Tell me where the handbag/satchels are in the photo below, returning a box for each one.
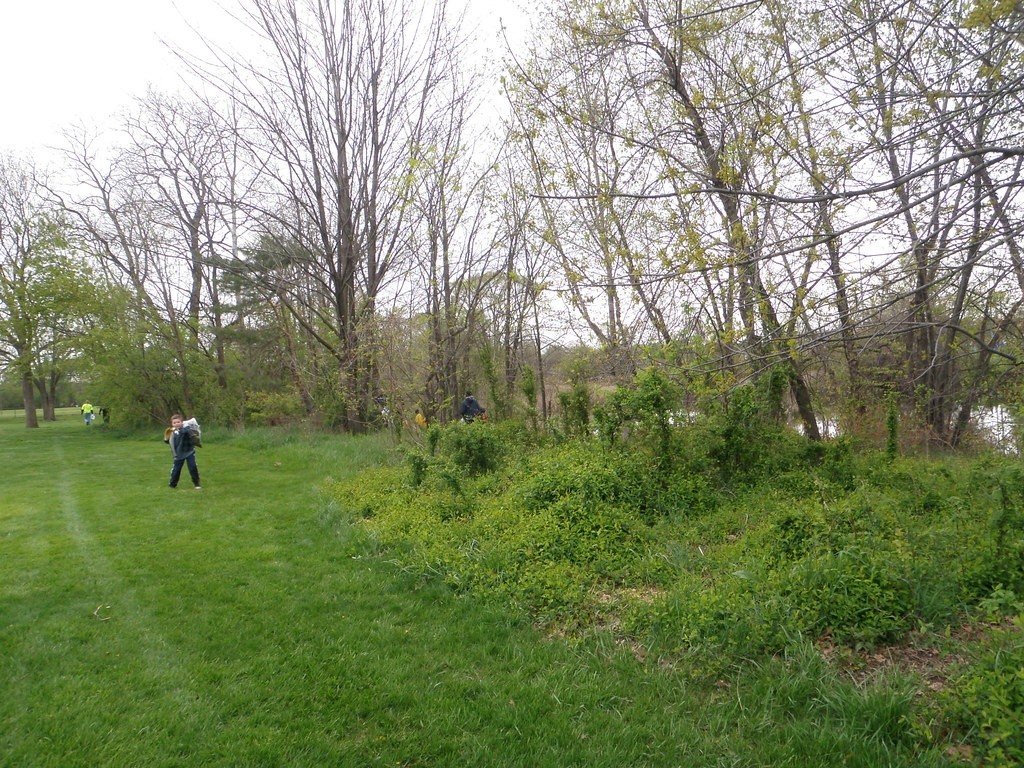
[91,414,95,420]
[183,418,202,448]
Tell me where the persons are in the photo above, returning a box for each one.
[415,408,426,425]
[81,400,95,425]
[98,403,111,423]
[377,389,388,407]
[165,413,202,490]
[459,391,486,424]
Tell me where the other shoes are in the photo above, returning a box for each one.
[87,422,89,425]
[195,486,201,489]
